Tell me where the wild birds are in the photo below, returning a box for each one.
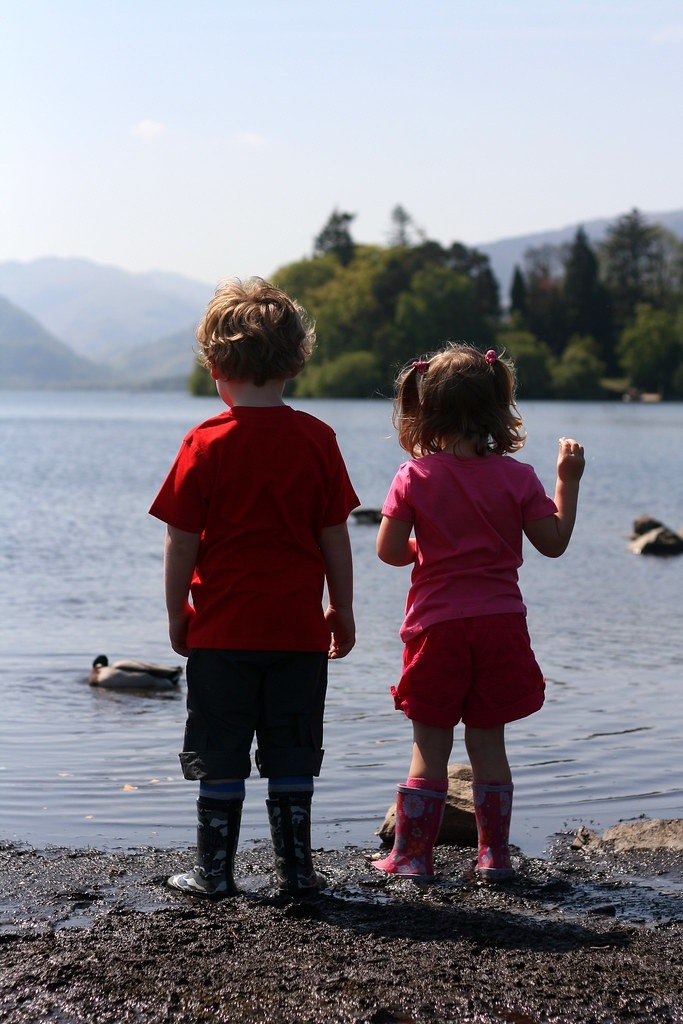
[90,655,184,690]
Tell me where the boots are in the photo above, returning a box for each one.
[372,783,446,882]
[166,799,243,898]
[469,781,514,883]
[266,796,327,893]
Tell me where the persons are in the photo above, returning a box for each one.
[148,278,360,900]
[366,346,585,884]
